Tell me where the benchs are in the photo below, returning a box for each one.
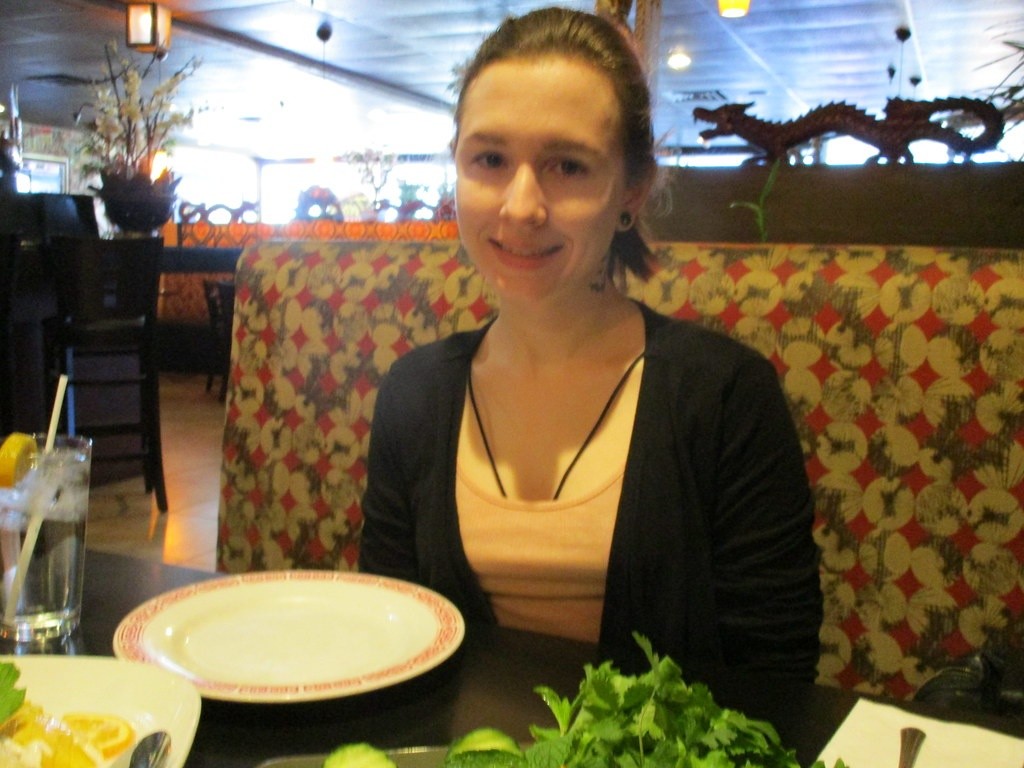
[217,232,1022,717]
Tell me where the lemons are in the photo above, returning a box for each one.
[0,704,133,768]
[0,432,38,489]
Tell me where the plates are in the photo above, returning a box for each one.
[113,568,464,704]
[1,654,202,768]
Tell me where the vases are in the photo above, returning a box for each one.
[86,168,182,237]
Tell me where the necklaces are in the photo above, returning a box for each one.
[464,353,647,505]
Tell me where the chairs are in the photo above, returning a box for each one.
[202,279,234,403]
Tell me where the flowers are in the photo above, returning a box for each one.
[74,37,205,176]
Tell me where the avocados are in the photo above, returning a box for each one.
[444,726,528,768]
[321,741,398,768]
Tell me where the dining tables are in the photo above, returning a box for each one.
[0,550,1024,767]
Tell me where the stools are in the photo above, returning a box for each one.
[44,238,171,512]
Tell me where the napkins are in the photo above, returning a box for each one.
[806,698,1024,768]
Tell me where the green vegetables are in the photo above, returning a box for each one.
[525,630,849,768]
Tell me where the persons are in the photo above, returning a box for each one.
[359,6,825,702]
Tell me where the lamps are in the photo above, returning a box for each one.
[718,1,750,17]
[126,4,172,55]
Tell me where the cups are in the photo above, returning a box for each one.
[0,433,94,641]
